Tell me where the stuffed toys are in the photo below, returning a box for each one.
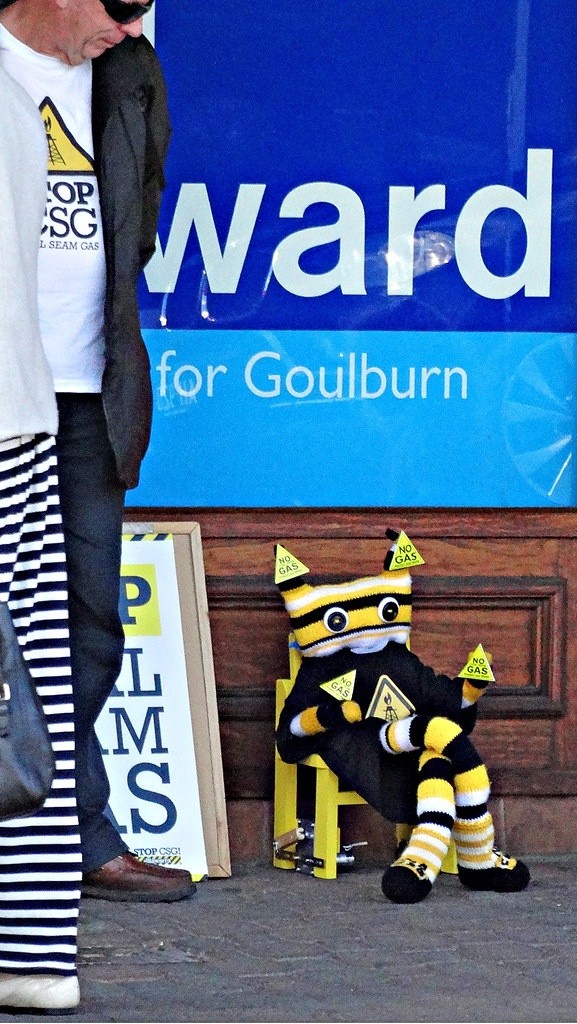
[274,530,533,905]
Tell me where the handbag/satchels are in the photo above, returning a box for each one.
[0,604,56,821]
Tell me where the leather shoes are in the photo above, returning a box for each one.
[80,850,195,905]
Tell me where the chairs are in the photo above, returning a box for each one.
[274,634,459,879]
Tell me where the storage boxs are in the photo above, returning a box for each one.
[94,521,233,882]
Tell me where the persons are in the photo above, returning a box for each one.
[0,48,87,1018]
[0,0,193,899]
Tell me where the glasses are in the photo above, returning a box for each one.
[100,0,152,24]
